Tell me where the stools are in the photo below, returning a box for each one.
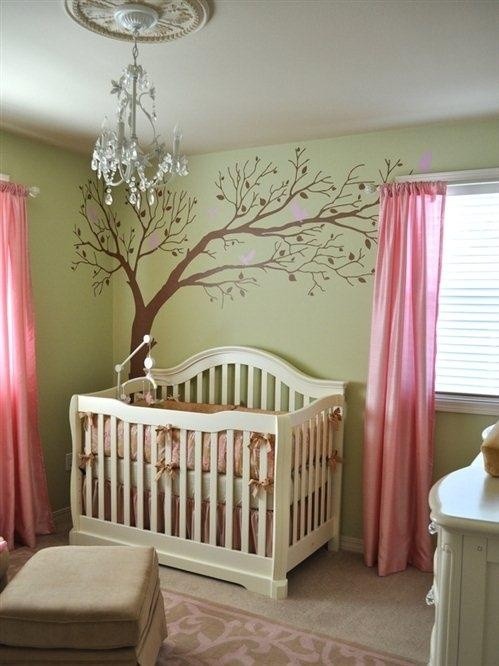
[0,545,169,665]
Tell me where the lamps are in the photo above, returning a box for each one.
[90,4,190,210]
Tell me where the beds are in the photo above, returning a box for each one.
[67,344,351,601]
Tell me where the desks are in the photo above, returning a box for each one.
[422,447,498,666]
